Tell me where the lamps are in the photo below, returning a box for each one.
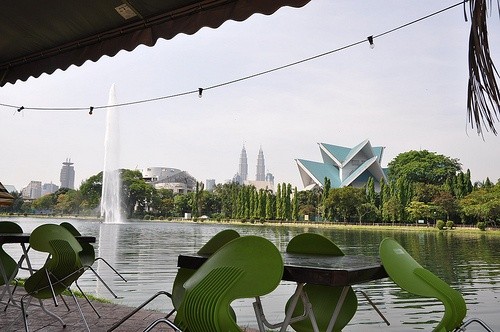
[110,1,143,23]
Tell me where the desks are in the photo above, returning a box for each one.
[177,254,391,332]
[0,232,96,318]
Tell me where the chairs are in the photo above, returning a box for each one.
[56,221,127,318]
[379,237,496,332]
[0,221,24,311]
[281,232,390,331]
[141,235,283,332]
[20,223,118,332]
[107,230,241,332]
